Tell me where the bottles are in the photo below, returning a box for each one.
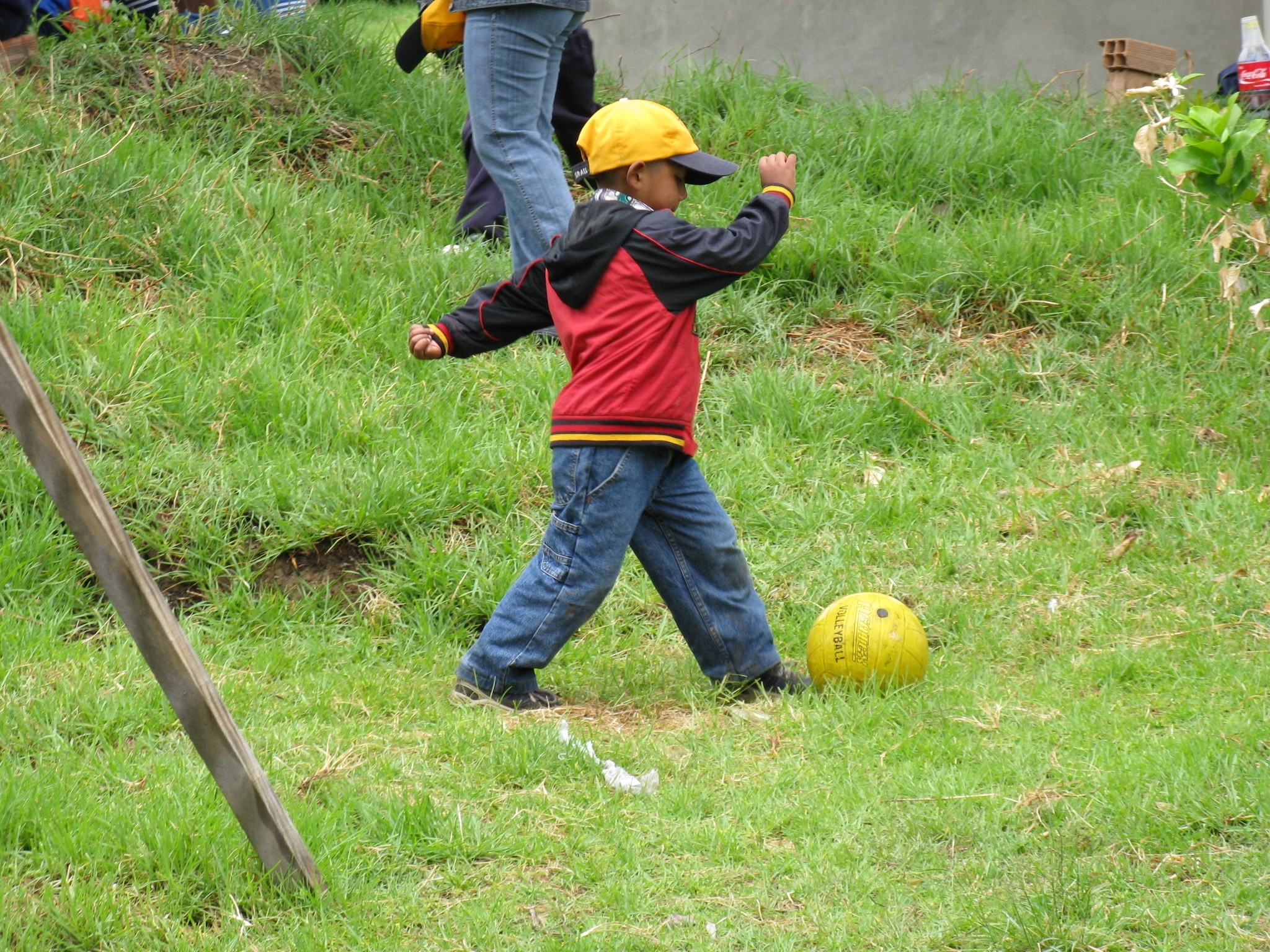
[1238,15,1269,122]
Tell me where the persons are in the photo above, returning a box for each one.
[0,0,421,44]
[407,95,822,715]
[449,0,590,351]
[444,23,600,253]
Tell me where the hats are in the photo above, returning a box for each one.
[571,98,738,185]
[395,0,466,73]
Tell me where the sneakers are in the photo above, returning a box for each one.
[448,676,561,713]
[711,662,812,705]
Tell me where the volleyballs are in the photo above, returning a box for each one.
[807,593,929,690]
